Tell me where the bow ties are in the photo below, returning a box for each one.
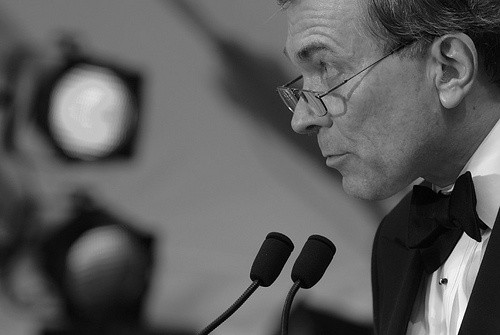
[407,171,482,273]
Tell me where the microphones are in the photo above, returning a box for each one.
[282,235,336,335]
[199,232,294,335]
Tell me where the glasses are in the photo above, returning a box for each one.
[275,37,417,117]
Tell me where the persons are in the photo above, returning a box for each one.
[276,0,500,335]
